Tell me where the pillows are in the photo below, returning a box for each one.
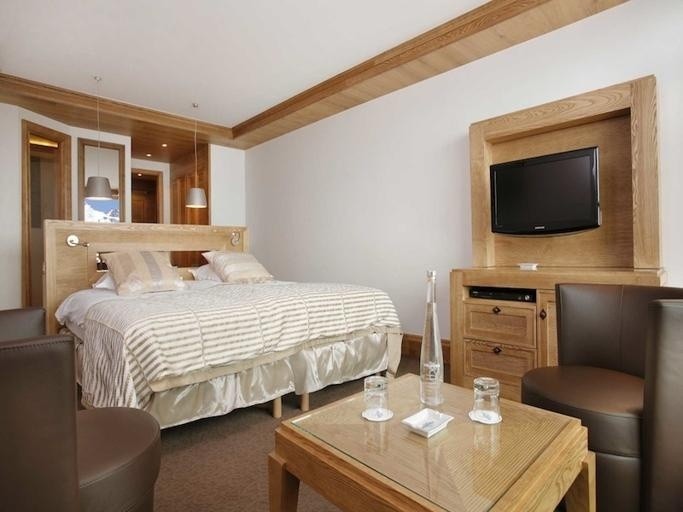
[201,250,274,283]
[99,250,184,297]
[92,271,183,290]
[187,263,273,282]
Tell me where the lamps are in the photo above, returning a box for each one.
[185,104,207,208]
[83,76,113,201]
[231,231,241,246]
[66,234,90,247]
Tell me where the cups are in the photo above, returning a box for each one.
[473,376,500,423]
[363,376,392,419]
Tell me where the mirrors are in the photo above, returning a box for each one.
[20,118,73,307]
[131,168,164,224]
[76,137,125,223]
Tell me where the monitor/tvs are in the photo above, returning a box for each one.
[490,146,600,238]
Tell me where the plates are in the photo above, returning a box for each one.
[400,407,454,437]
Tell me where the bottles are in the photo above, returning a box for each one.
[420,270,447,406]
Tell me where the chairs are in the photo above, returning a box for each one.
[521,283,683,512]
[0,308,161,512]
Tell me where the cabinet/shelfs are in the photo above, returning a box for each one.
[449,266,668,403]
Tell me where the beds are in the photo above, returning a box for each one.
[42,219,388,431]
